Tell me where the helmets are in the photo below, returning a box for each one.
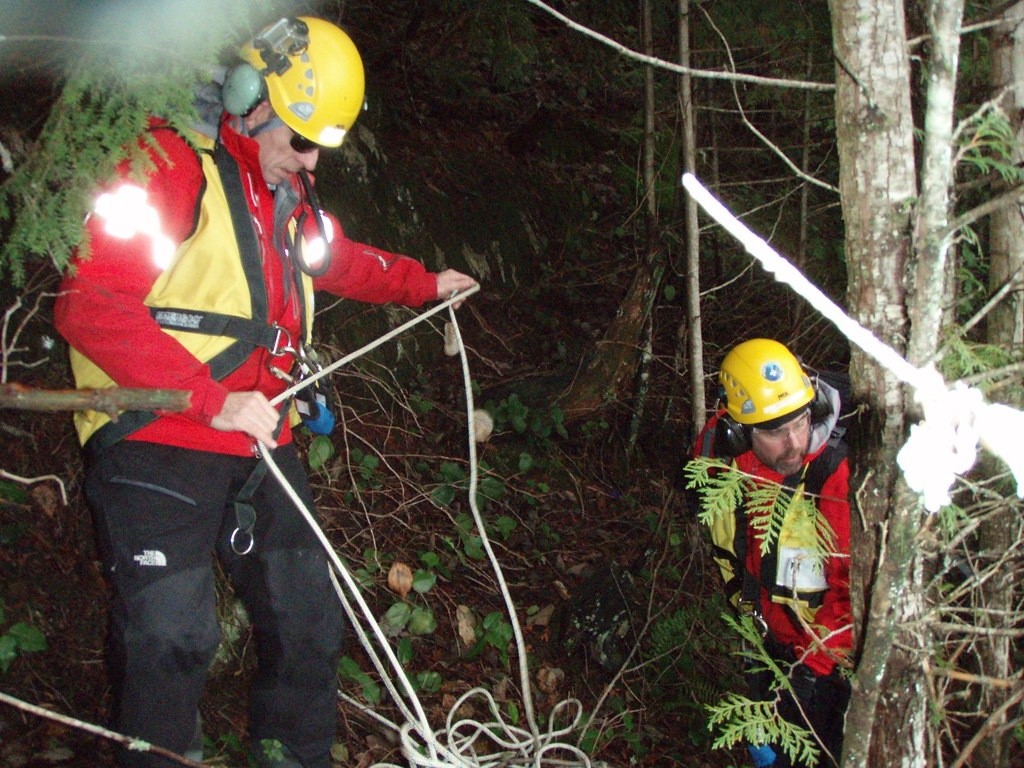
[719,338,815,424]
[237,16,364,148]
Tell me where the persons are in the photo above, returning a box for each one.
[686,336,854,768]
[51,16,478,768]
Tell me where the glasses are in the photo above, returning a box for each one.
[288,125,319,155]
[753,407,811,442]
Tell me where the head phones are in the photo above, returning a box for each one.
[222,65,268,118]
[716,365,831,456]
